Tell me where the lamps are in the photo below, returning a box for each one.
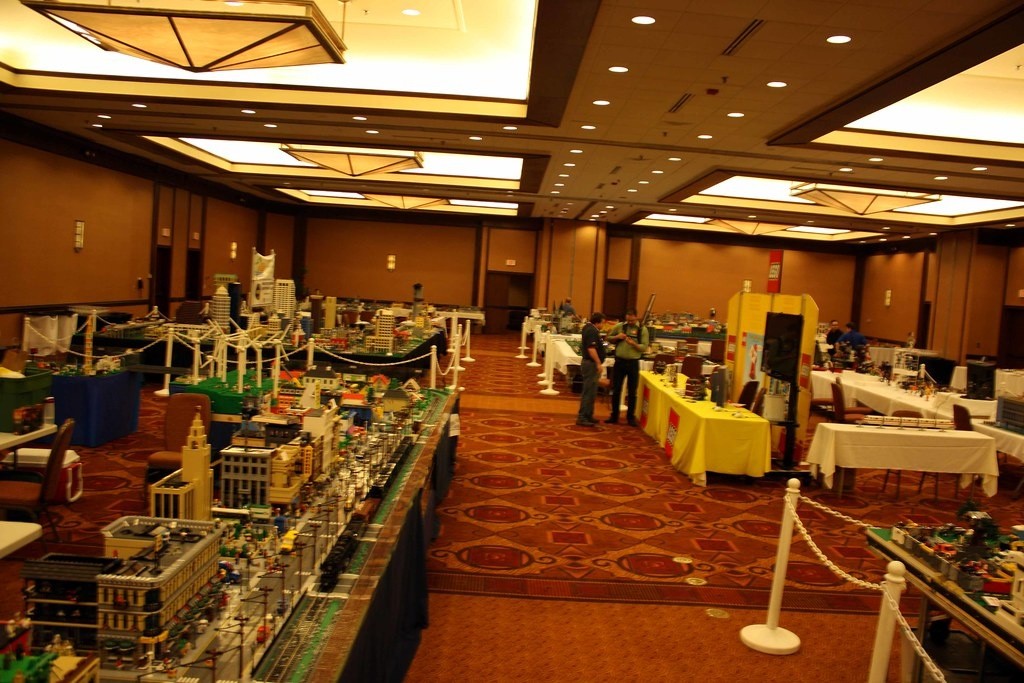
[358,194,449,211]
[742,279,752,292]
[387,254,396,272]
[18,0,349,73]
[278,143,423,178]
[73,220,84,253]
[884,288,892,307]
[790,181,942,215]
[701,218,797,236]
[230,241,237,262]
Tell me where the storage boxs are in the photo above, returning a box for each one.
[830,466,856,493]
[1,449,83,503]
[0,367,54,432]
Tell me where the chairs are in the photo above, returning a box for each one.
[952,404,1001,503]
[528,317,645,393]
[144,393,211,511]
[882,410,939,501]
[830,382,864,425]
[653,354,767,416]
[1,419,76,554]
[808,379,834,420]
[835,376,873,414]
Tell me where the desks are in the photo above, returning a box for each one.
[334,304,486,335]
[864,524,1024,683]
[0,521,43,562]
[805,367,1024,503]
[869,347,939,367]
[0,424,58,471]
[634,371,771,488]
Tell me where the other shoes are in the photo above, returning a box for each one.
[628,419,635,426]
[588,417,599,423]
[576,419,594,426]
[605,417,617,423]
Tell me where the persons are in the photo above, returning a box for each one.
[604,309,649,426]
[563,297,575,316]
[576,313,606,426]
[826,321,866,362]
